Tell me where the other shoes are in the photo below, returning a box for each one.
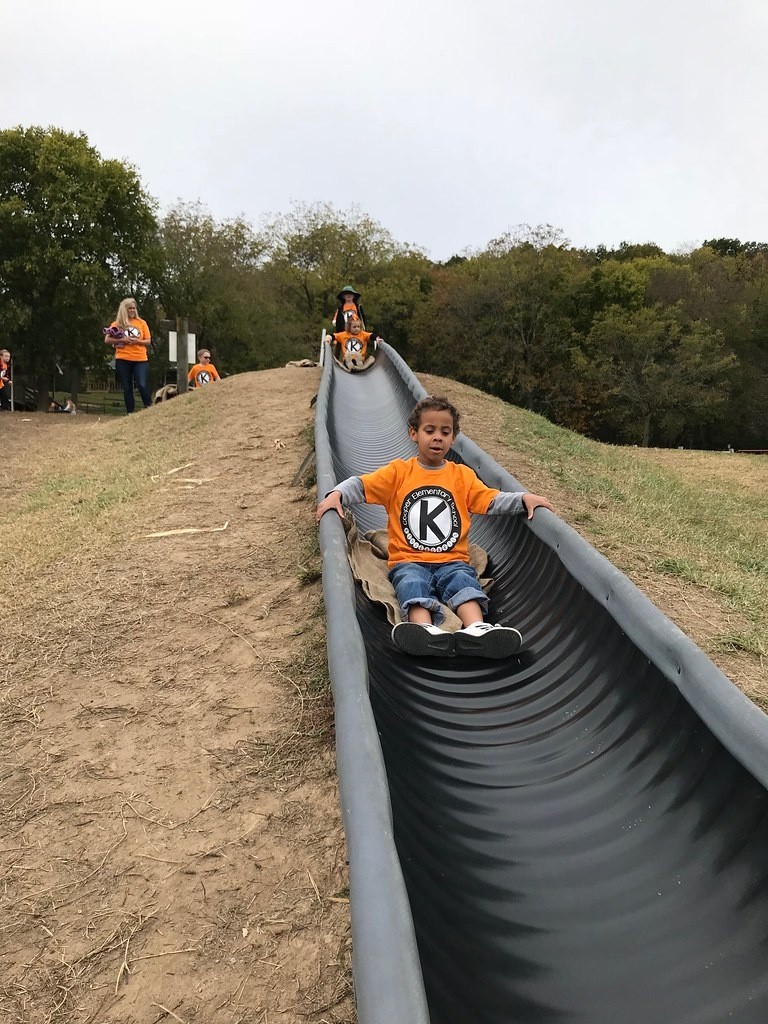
[355,353,363,366]
[346,354,353,368]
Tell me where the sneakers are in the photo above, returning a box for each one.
[391,622,453,657]
[450,622,522,658]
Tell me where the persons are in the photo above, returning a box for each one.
[0,349,13,411]
[104,298,154,416]
[322,286,384,371]
[315,396,556,658]
[188,349,221,387]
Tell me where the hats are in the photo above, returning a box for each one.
[337,286,361,301]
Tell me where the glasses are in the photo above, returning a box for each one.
[202,357,211,359]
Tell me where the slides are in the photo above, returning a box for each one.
[314,339,768,1024]
